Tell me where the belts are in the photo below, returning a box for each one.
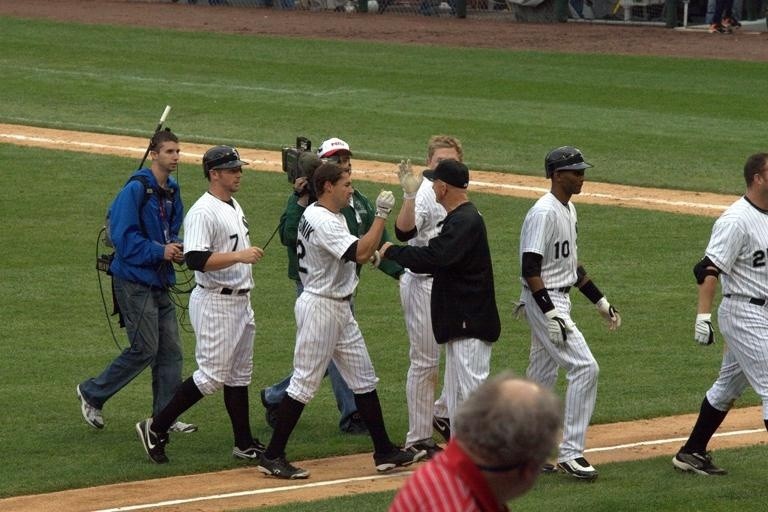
[550,286,576,294]
[345,293,354,301]
[727,292,765,307]
[198,286,248,296]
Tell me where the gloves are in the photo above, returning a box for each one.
[694,312,713,347]
[373,190,395,220]
[595,298,621,332]
[545,309,574,350]
[396,157,424,199]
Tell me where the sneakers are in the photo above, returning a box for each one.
[672,448,728,477]
[559,457,598,480]
[133,418,170,466]
[228,444,268,459]
[344,417,371,434]
[258,389,281,428]
[432,413,452,440]
[543,463,556,475]
[368,447,428,473]
[722,16,743,33]
[405,439,444,463]
[258,453,311,481]
[707,20,731,37]
[165,423,197,439]
[76,383,103,430]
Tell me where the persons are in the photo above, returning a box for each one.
[387,369,566,512]
[708,0,742,35]
[399,135,465,461]
[76,126,198,436]
[670,152,768,476]
[134,144,267,466]
[261,135,405,433]
[257,163,427,479]
[380,159,502,441]
[522,144,623,481]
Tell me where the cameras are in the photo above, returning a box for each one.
[283,137,356,211]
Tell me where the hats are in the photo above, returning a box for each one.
[546,145,594,178]
[202,145,250,173]
[317,137,354,160]
[423,157,469,191]
